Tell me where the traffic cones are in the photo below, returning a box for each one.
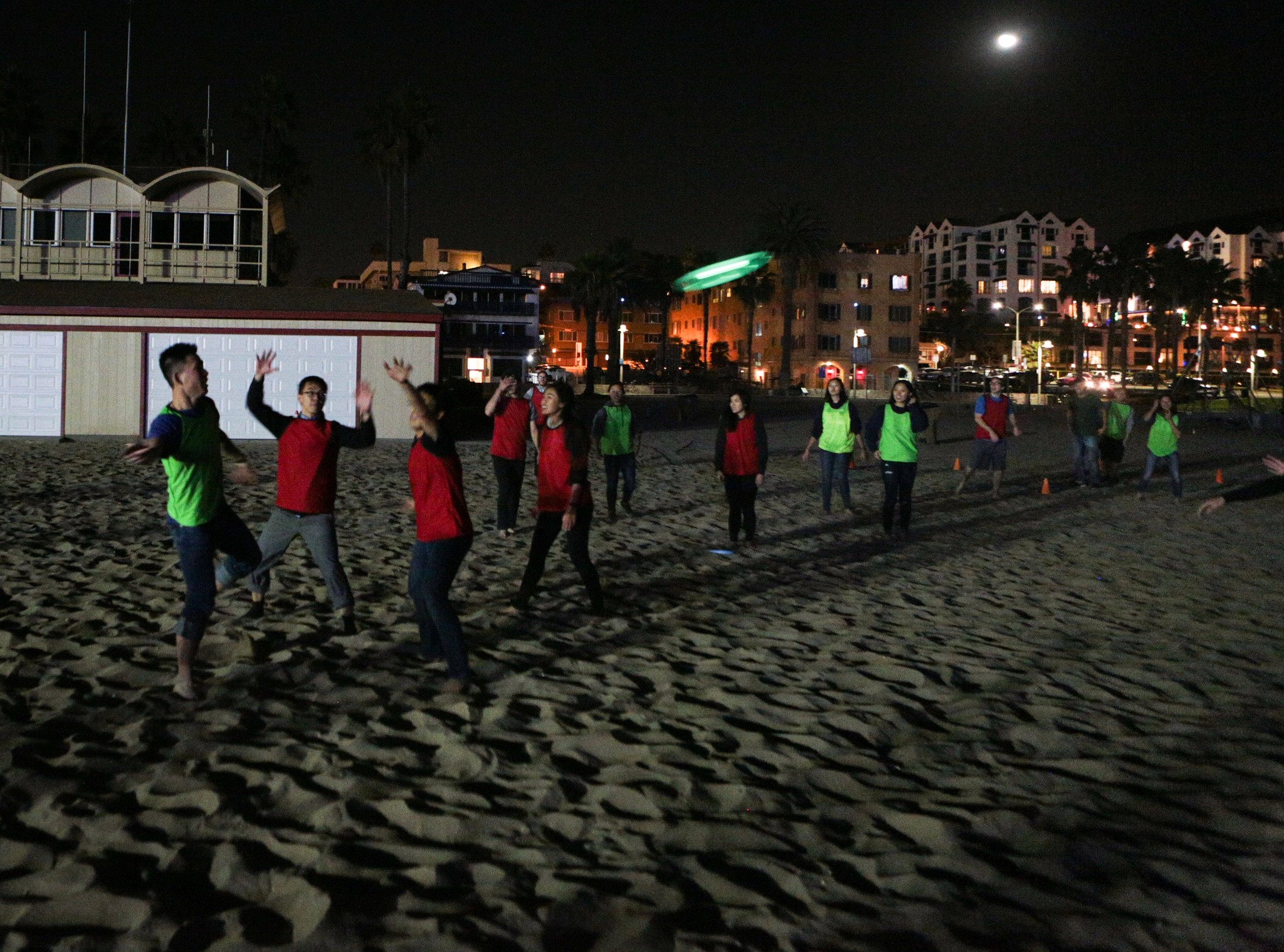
[848,456,857,469]
[1040,479,1051,495]
[953,458,960,471]
[1216,468,1224,484]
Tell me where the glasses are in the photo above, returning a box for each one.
[302,392,325,396]
[538,376,546,378]
[992,382,1001,385]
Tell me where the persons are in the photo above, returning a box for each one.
[800,378,868,515]
[1134,393,1183,509]
[713,389,770,544]
[1066,377,1107,490]
[951,375,1022,500]
[123,342,263,700]
[383,356,474,695]
[1197,453,1284,519]
[865,381,930,538]
[510,381,607,615]
[1103,387,1135,485]
[240,349,377,634]
[592,382,636,525]
[484,372,541,540]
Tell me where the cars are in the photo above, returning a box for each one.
[1056,369,1162,387]
[529,365,575,384]
[918,366,1056,392]
[1165,376,1221,400]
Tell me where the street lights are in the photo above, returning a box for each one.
[1198,305,1223,363]
[1231,301,1242,326]
[992,303,1043,364]
